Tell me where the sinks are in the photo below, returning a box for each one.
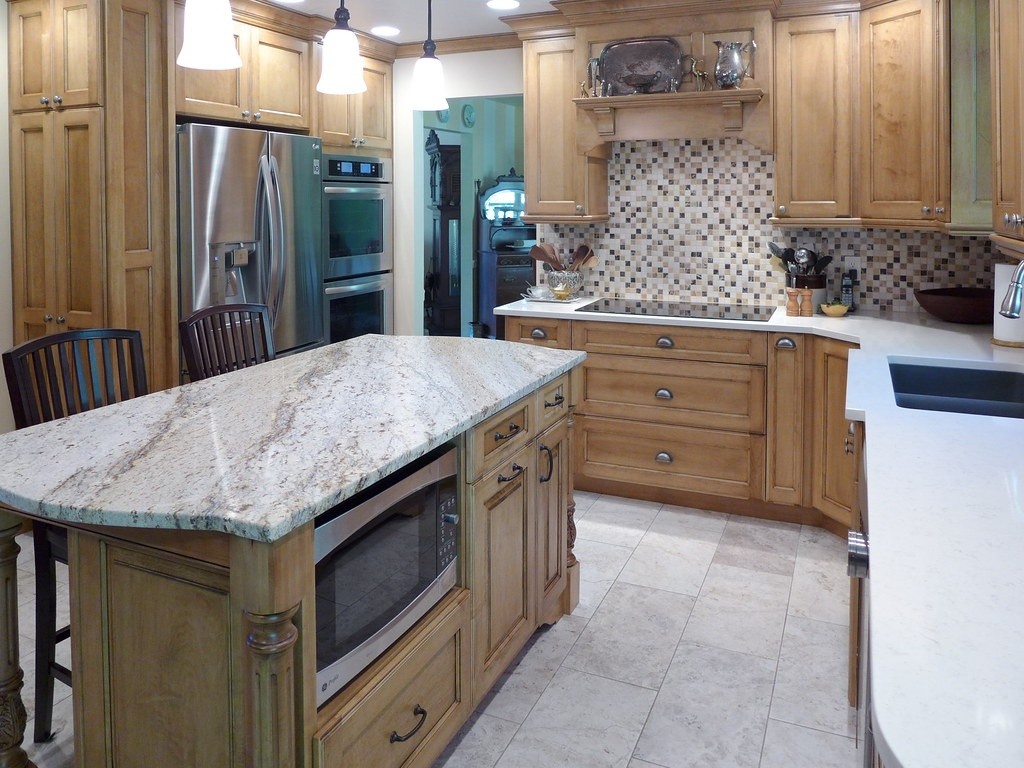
[889,363,1024,419]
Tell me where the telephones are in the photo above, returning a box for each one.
[841,273,857,312]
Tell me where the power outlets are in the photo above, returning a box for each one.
[845,257,862,281]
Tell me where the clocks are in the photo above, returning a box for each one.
[463,105,475,127]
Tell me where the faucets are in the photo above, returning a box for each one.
[999,258,1024,319]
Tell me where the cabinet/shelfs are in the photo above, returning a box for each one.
[425,130,461,337]
[497,0,1024,262]
[309,14,396,156]
[165,1,311,132]
[476,169,537,340]
[67,314,864,768]
[7,0,179,421]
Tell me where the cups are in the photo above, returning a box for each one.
[527,287,547,298]
[785,273,826,313]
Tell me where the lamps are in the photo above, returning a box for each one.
[316,0,367,94]
[176,0,243,70]
[404,0,449,111]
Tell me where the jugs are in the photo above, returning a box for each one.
[713,40,757,89]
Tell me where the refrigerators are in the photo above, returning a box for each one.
[177,122,326,386]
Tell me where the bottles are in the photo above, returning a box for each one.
[843,276,852,307]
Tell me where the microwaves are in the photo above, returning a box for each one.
[313,446,459,709]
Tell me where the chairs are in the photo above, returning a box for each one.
[1,328,147,744]
[178,303,275,382]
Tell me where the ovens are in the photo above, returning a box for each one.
[322,153,394,344]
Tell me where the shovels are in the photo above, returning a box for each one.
[568,243,599,270]
[539,244,560,262]
[528,245,563,270]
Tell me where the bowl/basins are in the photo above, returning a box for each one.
[914,288,994,324]
[820,304,849,317]
[547,271,584,299]
